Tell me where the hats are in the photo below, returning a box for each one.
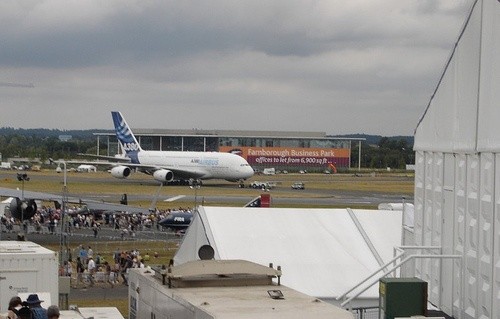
[23,294,44,305]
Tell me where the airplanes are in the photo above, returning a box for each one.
[157,193,270,231]
[50,112,254,186]
[1,185,128,220]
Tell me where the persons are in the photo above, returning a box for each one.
[6,297,23,319]
[17,307,33,319]
[1,199,193,289]
[22,294,48,319]
[46,305,60,319]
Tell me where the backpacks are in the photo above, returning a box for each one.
[8,307,31,319]
